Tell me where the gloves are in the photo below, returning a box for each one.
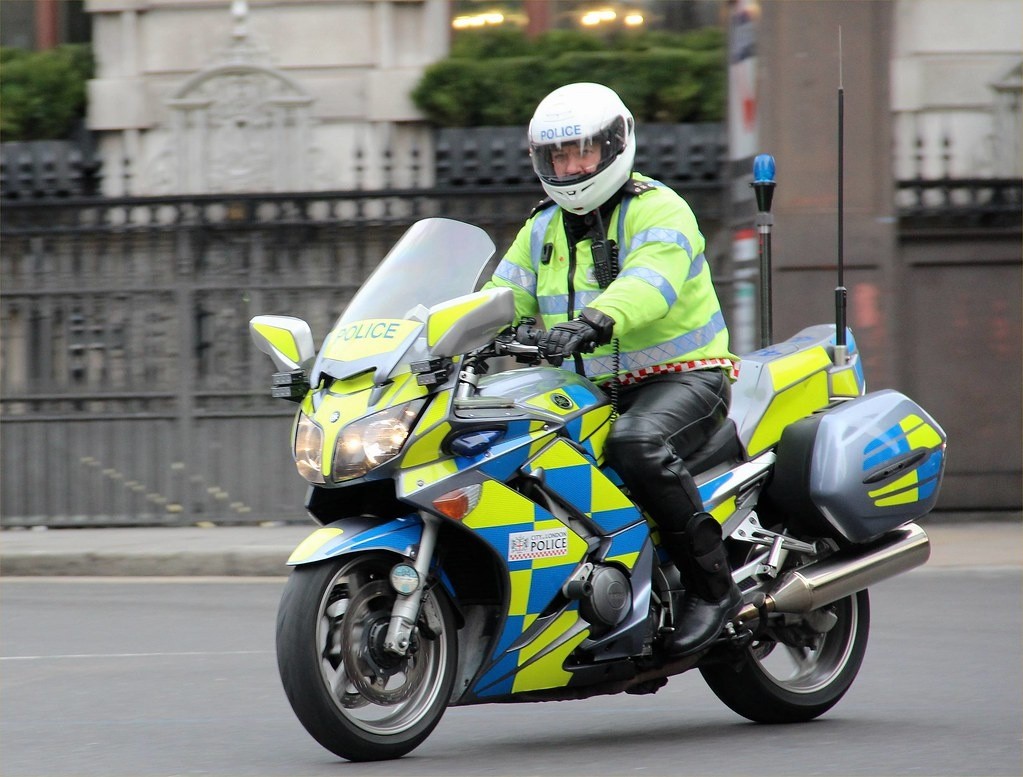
[538,315,597,366]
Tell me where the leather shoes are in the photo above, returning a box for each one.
[670,576,745,655]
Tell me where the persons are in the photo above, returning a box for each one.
[479,82,742,662]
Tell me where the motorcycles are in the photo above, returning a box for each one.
[249,217,947,763]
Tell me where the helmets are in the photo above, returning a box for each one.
[528,83,636,216]
